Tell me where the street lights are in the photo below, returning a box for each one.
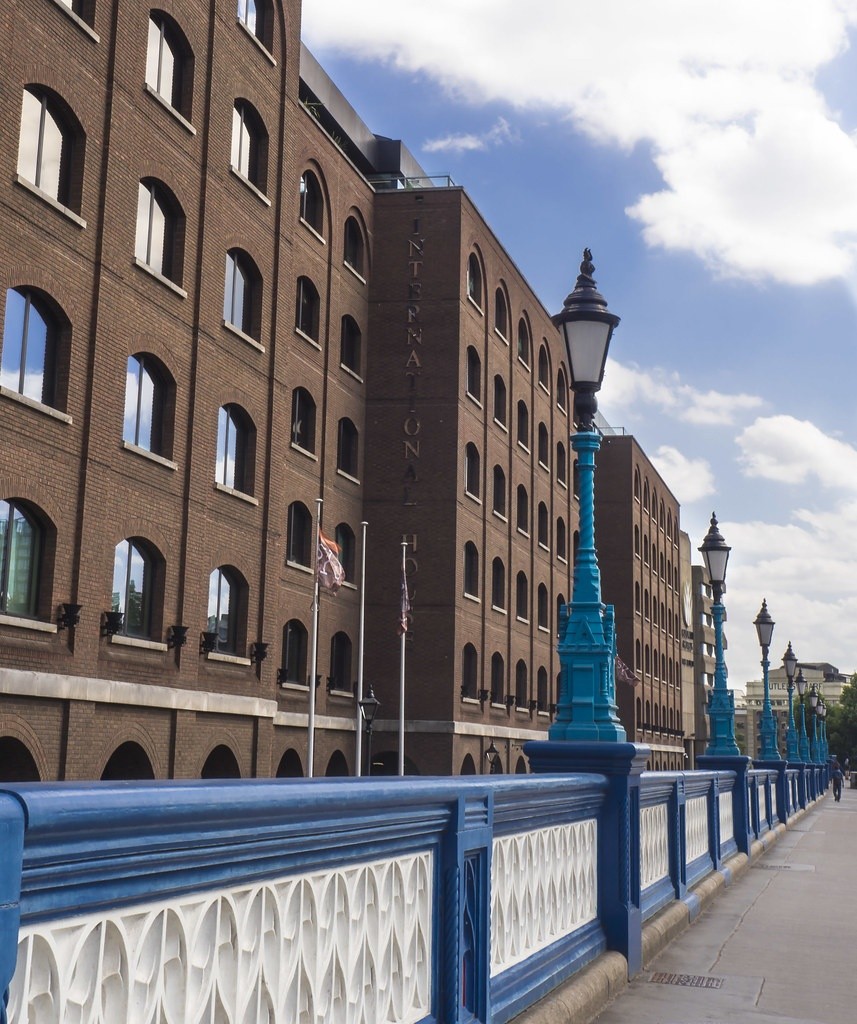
[814,697,827,764]
[752,597,783,760]
[808,684,822,764]
[793,667,813,764]
[697,512,742,757]
[547,247,632,745]
[819,702,830,761]
[781,640,803,762]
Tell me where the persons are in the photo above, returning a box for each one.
[831,758,852,802]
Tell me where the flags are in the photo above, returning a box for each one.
[315,523,346,597]
[614,654,641,687]
[399,555,411,634]
